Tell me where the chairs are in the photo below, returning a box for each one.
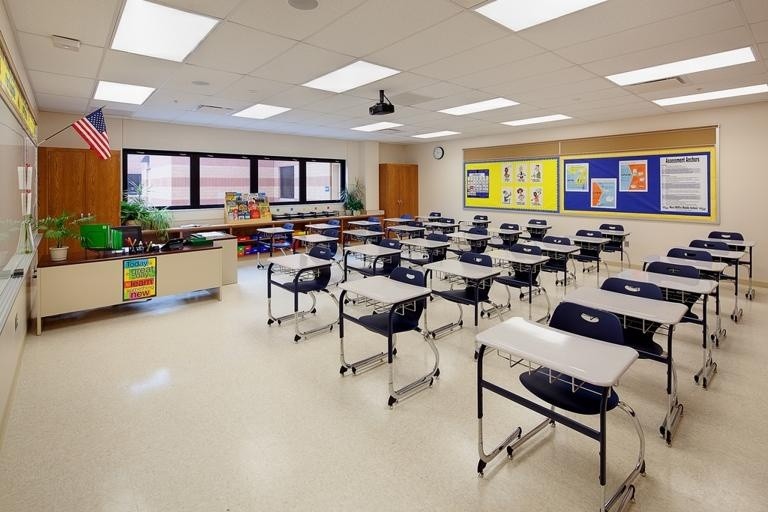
[575,230,603,273]
[359,239,401,306]
[428,212,442,223]
[277,246,340,335]
[506,301,645,512]
[526,220,552,241]
[595,278,677,435]
[352,267,440,409]
[401,233,448,267]
[599,223,630,270]
[383,215,414,238]
[452,228,487,255]
[367,217,380,228]
[645,262,710,382]
[440,253,504,339]
[494,245,551,324]
[472,215,488,227]
[442,218,455,235]
[689,240,739,319]
[667,248,718,339]
[259,223,295,256]
[311,228,344,285]
[539,235,577,286]
[708,231,752,298]
[322,219,341,237]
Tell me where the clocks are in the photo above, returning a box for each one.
[432,147,444,160]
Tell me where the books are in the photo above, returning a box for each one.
[227,192,270,219]
[80,224,122,251]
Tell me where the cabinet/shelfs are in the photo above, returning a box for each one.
[37,146,122,255]
[379,163,419,238]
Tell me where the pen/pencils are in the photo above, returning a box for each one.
[125,237,137,248]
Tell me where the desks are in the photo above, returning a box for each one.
[699,238,755,300]
[387,225,425,238]
[643,256,727,349]
[343,229,385,261]
[596,230,630,269]
[36,243,223,335]
[486,228,522,266]
[399,238,451,267]
[422,254,501,360]
[267,253,340,343]
[458,220,491,232]
[524,224,552,242]
[292,234,340,255]
[474,317,645,512]
[522,240,581,287]
[256,227,295,269]
[666,247,745,322]
[610,269,720,388]
[347,220,381,230]
[560,284,689,447]
[482,249,551,323]
[422,222,460,234]
[567,235,611,285]
[415,215,442,222]
[189,231,238,286]
[338,276,432,405]
[344,244,403,304]
[445,232,491,257]
[305,223,341,235]
[381,217,415,237]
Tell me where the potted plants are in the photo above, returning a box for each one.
[340,191,353,216]
[122,200,149,227]
[349,194,365,216]
[32,209,85,261]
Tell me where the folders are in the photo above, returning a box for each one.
[80,223,122,249]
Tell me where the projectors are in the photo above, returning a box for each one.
[369,102,394,115]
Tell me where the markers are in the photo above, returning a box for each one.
[146,241,153,251]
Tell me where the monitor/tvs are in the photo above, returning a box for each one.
[111,225,141,248]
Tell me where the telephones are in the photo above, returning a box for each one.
[186,233,206,244]
[162,237,184,252]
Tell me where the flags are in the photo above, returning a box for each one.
[73,105,112,160]
